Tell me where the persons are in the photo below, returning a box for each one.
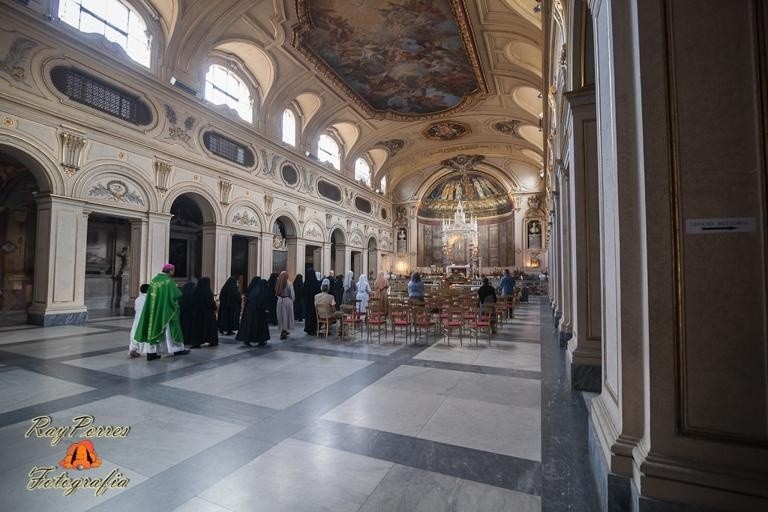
[478,278,496,334]
[500,269,515,318]
[407,272,424,306]
[127,263,390,361]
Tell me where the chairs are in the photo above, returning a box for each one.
[311,277,526,346]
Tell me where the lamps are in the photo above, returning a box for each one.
[438,182,477,249]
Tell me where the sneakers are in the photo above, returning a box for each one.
[174,350,191,356]
[147,353,161,360]
[129,351,140,358]
